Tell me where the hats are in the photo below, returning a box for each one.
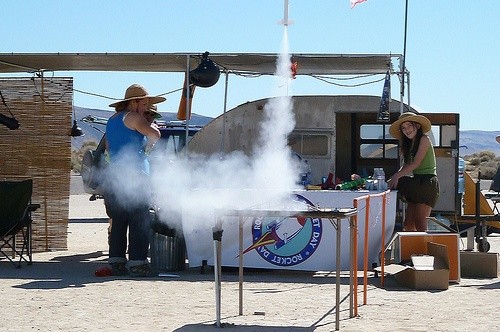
[145,104,162,118]
[389,112,432,139]
[108,85,166,108]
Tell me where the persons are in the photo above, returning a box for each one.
[388,112,440,232]
[105,82,161,277]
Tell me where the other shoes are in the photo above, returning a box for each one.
[110,263,129,276]
[400,259,415,268]
[130,265,159,278]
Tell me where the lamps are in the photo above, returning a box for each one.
[69,105,85,140]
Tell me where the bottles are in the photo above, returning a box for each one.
[458,157,464,194]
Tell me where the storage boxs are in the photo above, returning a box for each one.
[373,241,450,291]
[384,231,461,283]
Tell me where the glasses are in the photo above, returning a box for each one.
[146,112,155,117]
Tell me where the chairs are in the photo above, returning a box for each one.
[0,179,41,269]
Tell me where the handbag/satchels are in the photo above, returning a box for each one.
[82,149,112,191]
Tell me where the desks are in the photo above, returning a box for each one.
[211,207,358,331]
[180,186,399,276]
